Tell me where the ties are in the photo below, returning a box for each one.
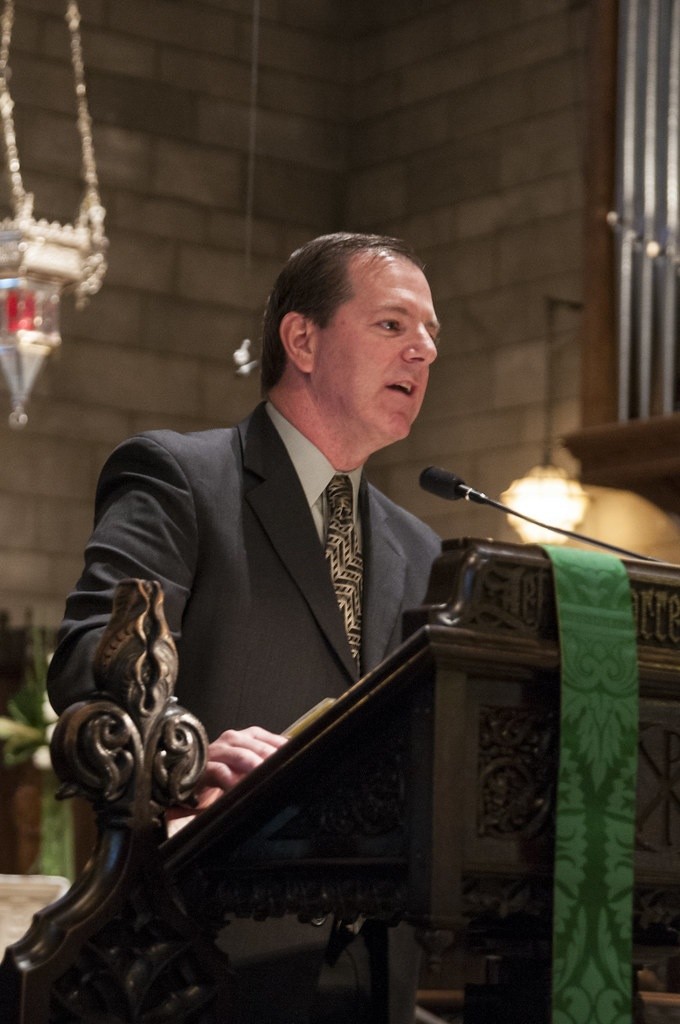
[324,476,364,674]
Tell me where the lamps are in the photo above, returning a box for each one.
[0,0,111,433]
[501,293,592,551]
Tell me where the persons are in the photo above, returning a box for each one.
[45,233,487,1024]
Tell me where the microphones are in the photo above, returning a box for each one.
[420,465,661,563]
